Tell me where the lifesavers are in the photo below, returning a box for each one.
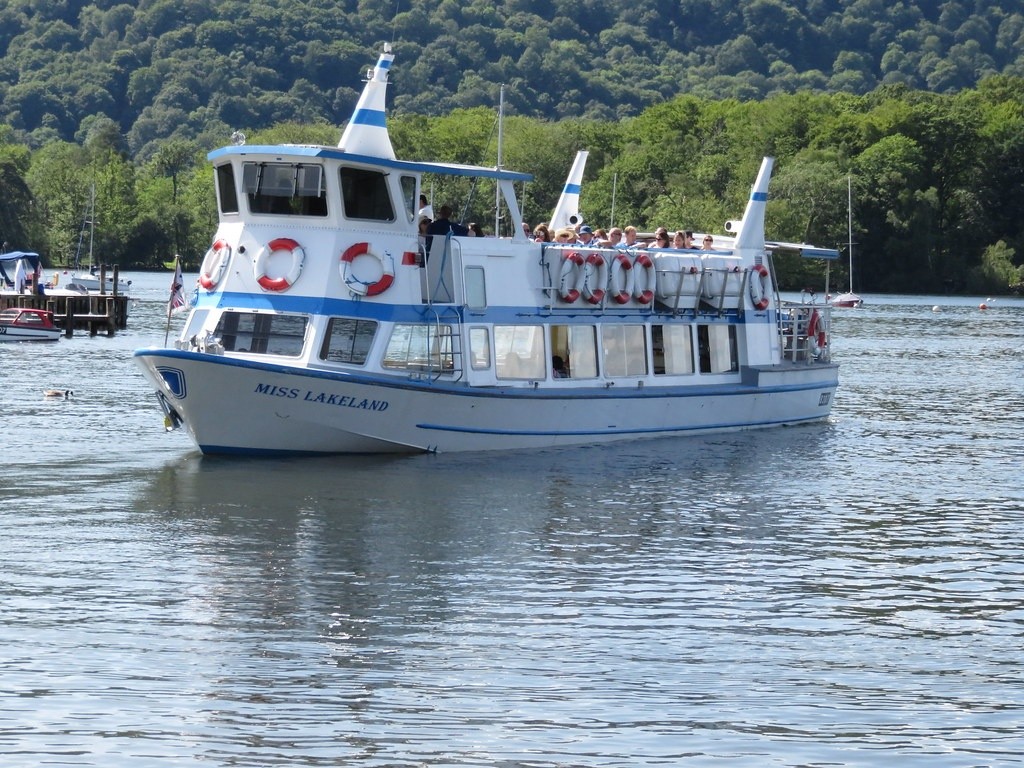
[254,236,306,290]
[559,252,657,304]
[199,238,231,288]
[338,241,396,296]
[807,313,828,355]
[750,265,771,310]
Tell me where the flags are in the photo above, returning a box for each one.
[168,261,190,318]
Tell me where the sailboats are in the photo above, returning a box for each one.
[833,175,864,307]
[70,183,133,292]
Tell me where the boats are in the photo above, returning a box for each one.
[0,251,55,291]
[0,307,61,342]
[130,42,840,464]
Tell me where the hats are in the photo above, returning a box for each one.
[579,226,591,235]
[437,205,452,215]
[555,228,573,241]
[686,232,694,240]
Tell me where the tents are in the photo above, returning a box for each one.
[0,251,40,288]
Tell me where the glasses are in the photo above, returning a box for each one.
[612,232,622,236]
[593,235,601,239]
[655,237,663,241]
[704,239,713,242]
[524,229,529,232]
[561,234,569,237]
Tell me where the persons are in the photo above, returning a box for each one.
[14,259,27,294]
[519,223,717,251]
[419,194,485,238]
[498,352,570,378]
